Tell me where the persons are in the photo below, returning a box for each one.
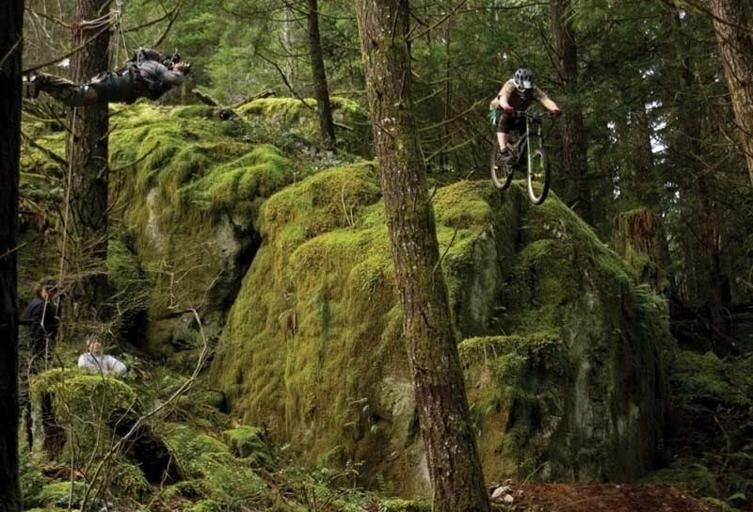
[489,67,561,167]
[78,336,127,378]
[23,284,60,368]
[23,48,190,106]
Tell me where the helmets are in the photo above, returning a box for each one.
[513,68,533,89]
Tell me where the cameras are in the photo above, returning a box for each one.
[179,61,194,71]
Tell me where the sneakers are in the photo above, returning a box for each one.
[22,72,40,98]
[500,149,513,161]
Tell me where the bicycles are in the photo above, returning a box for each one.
[487,107,561,205]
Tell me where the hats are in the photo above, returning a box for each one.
[40,276,56,288]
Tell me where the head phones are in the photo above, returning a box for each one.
[163,47,179,69]
